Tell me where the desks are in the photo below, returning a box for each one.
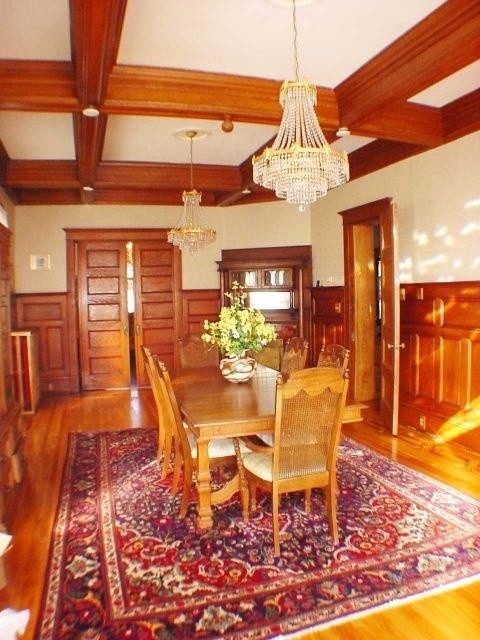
[167,361,368,531]
[10,325,40,415]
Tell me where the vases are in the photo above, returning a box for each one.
[219,356,258,385]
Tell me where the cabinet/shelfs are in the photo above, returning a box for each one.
[215,259,305,349]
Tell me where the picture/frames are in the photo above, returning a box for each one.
[30,254,51,270]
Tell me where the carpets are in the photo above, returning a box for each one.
[33,427,480,640]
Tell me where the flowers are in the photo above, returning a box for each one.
[201,281,279,360]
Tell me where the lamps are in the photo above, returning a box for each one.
[167,131,217,255]
[252,0,350,210]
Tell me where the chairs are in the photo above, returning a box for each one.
[280,337,310,381]
[237,367,349,557]
[140,344,188,465]
[316,344,351,368]
[174,336,219,371]
[151,355,252,519]
